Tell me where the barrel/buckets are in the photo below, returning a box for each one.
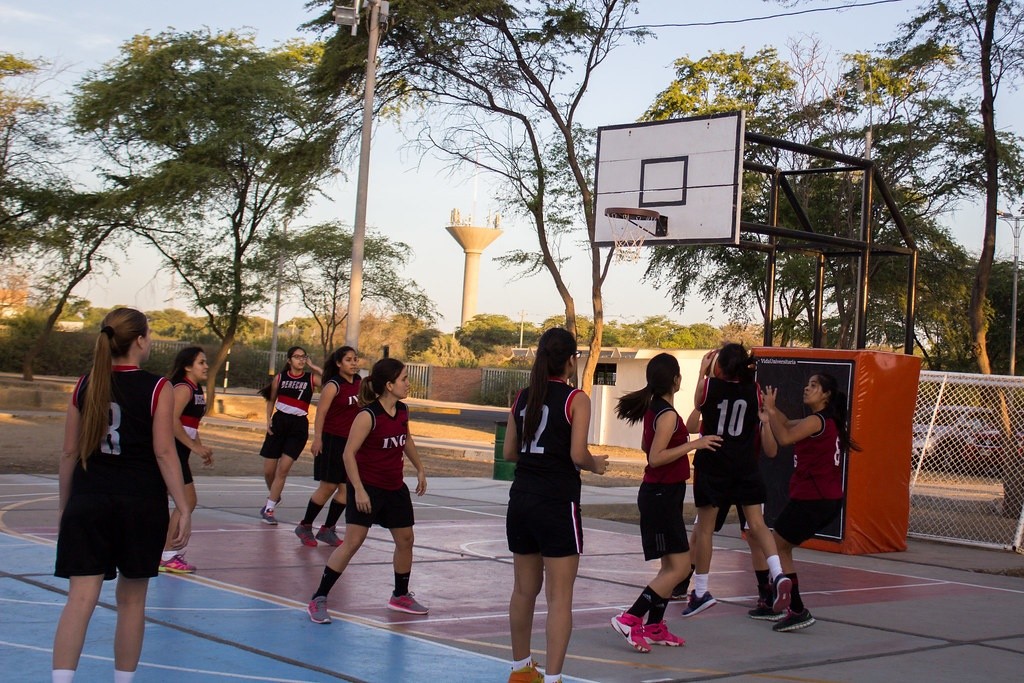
[492,421,517,480]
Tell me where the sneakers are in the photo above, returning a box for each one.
[263,509,278,525]
[772,608,816,632]
[260,497,282,515]
[295,520,318,546]
[642,620,685,647]
[387,591,429,614]
[671,588,686,600]
[612,610,650,652]
[772,574,792,613]
[682,589,716,617]
[747,603,784,621]
[306,593,332,623]
[508,662,544,683]
[158,551,197,573]
[315,525,342,546]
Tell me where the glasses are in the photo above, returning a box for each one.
[574,350,582,358]
[292,354,308,360]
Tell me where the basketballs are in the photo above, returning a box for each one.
[704,349,727,380]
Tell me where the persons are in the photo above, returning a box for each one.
[295,346,429,623]
[256,347,323,525]
[502,328,610,683]
[52,308,214,683]
[611,343,865,652]
